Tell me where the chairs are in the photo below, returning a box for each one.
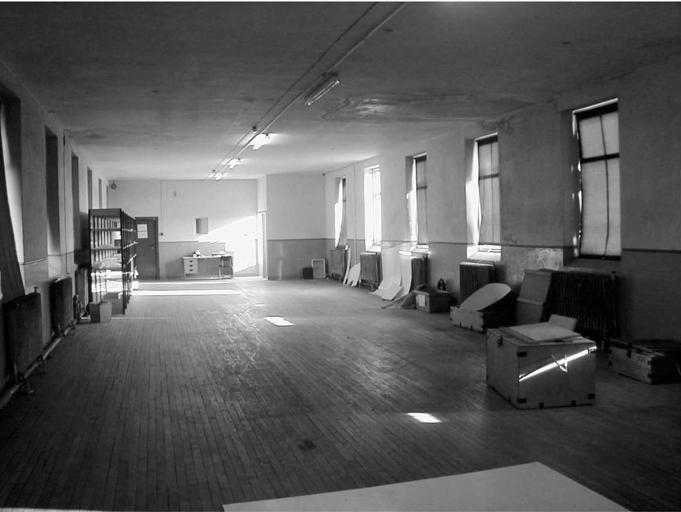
[216,256,233,280]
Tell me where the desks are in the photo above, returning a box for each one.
[182,256,232,281]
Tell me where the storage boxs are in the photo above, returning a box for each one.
[447,303,486,332]
[414,287,451,312]
[88,301,113,324]
[517,268,565,325]
[607,341,678,383]
[482,327,598,410]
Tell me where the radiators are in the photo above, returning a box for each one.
[457,261,498,305]
[1,293,46,392]
[76,266,91,314]
[411,256,428,289]
[541,264,620,346]
[358,252,380,292]
[327,249,349,280]
[48,274,77,340]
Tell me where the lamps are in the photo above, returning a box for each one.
[228,156,240,170]
[303,70,339,107]
[213,172,225,181]
[252,133,273,152]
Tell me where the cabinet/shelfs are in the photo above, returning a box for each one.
[88,204,138,314]
